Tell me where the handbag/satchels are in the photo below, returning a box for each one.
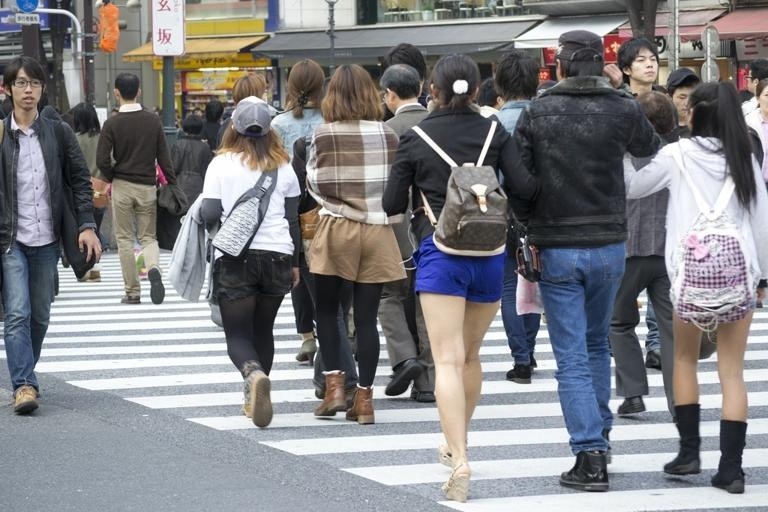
[515,235,543,284]
[211,156,278,262]
[89,177,110,210]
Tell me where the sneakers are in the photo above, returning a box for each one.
[410,385,437,404]
[148,267,165,305]
[644,348,662,371]
[120,295,141,304]
[314,384,358,411]
[616,395,646,414]
[506,351,537,385]
[13,385,38,413]
[384,359,423,395]
[77,263,101,282]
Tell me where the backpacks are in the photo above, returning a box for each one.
[410,120,510,258]
[668,143,762,333]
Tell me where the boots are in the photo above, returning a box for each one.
[346,382,375,426]
[663,403,703,477]
[710,419,747,495]
[239,359,274,428]
[559,426,614,492]
[313,369,349,417]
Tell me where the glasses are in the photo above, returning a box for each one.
[9,78,43,89]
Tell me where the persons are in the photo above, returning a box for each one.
[0,54,102,414]
[489,28,768,494]
[201,42,543,503]
[168,100,227,216]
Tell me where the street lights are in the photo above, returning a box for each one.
[94,0,144,119]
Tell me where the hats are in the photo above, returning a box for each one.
[554,30,604,63]
[665,68,700,92]
[230,95,273,138]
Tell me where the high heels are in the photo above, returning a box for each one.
[438,445,468,469]
[440,462,471,503]
[295,338,317,366]
